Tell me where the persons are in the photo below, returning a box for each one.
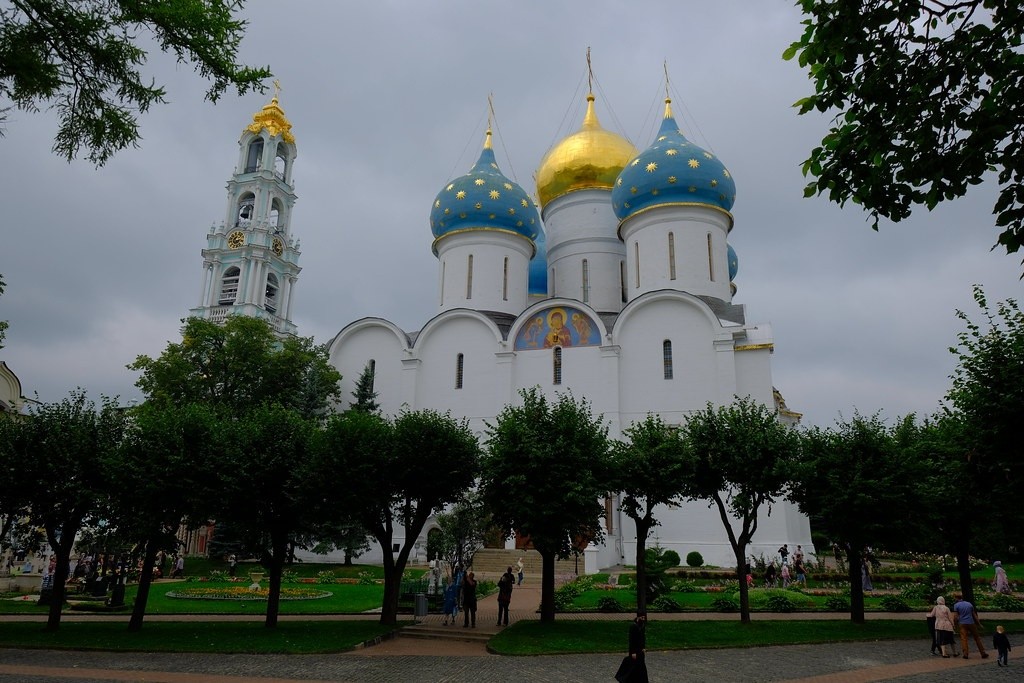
[21,537,184,595]
[501,566,516,611]
[228,554,236,579]
[993,625,1011,666]
[860,557,874,598]
[833,543,846,573]
[462,572,477,629]
[496,573,513,627]
[927,607,942,656]
[992,561,1012,596]
[517,558,524,585]
[926,596,960,659]
[443,577,457,626]
[951,591,989,659]
[745,543,807,591]
[453,564,467,611]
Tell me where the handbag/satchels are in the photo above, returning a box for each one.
[514,565,520,571]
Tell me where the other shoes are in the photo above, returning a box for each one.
[471,625,475,628]
[496,623,501,626]
[502,623,508,626]
[442,621,447,626]
[450,620,455,625]
[463,625,468,628]
